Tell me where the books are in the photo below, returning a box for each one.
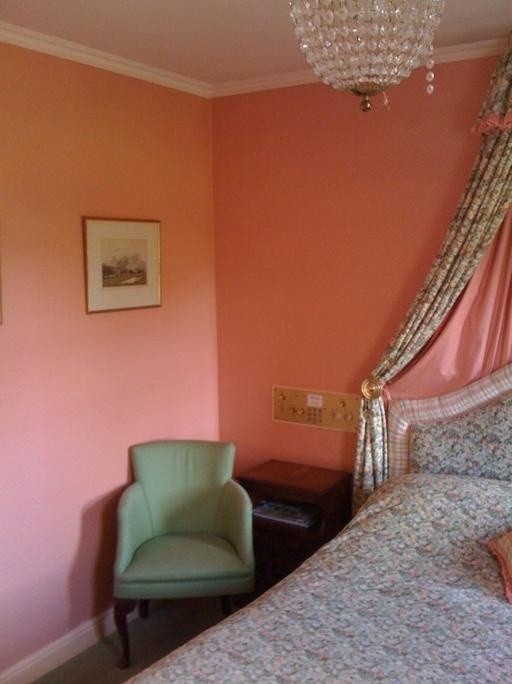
[253,500,319,528]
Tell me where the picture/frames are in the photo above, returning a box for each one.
[81,213,162,317]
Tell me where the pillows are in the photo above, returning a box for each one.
[484,526,512,603]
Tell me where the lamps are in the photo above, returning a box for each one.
[289,0,439,117]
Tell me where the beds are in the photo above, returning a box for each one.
[118,361,511,684]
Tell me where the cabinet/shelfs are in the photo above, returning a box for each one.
[239,458,354,553]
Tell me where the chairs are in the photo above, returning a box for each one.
[113,438,257,671]
